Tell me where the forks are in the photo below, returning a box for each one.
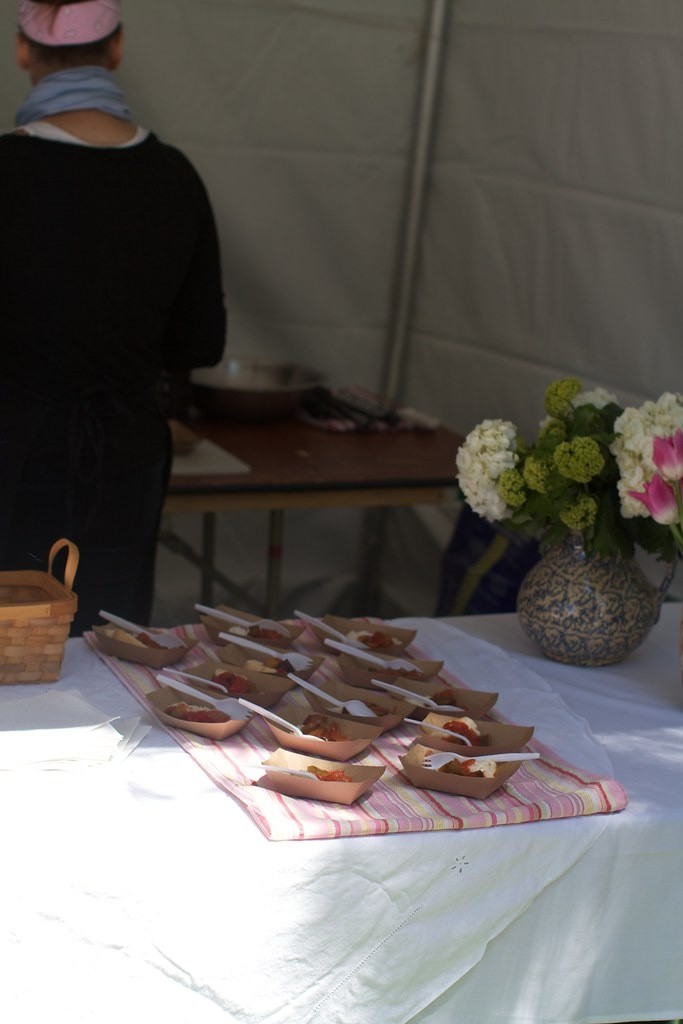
[219,632,314,672]
[421,752,540,771]
[194,604,291,639]
[155,674,254,721]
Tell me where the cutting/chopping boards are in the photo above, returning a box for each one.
[170,423,250,476]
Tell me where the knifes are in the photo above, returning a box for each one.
[337,392,440,431]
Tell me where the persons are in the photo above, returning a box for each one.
[0,1,230,639]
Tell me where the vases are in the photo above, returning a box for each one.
[518,524,678,668]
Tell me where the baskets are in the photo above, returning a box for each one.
[0,539,79,685]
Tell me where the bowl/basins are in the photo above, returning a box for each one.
[188,354,326,419]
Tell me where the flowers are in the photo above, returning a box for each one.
[456,376,683,571]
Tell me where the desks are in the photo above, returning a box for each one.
[0,602,683,1024]
[162,405,466,619]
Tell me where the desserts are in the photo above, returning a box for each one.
[107,624,498,783]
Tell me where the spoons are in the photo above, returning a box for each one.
[370,678,464,712]
[98,610,187,650]
[293,609,369,649]
[286,672,378,718]
[324,638,423,674]
